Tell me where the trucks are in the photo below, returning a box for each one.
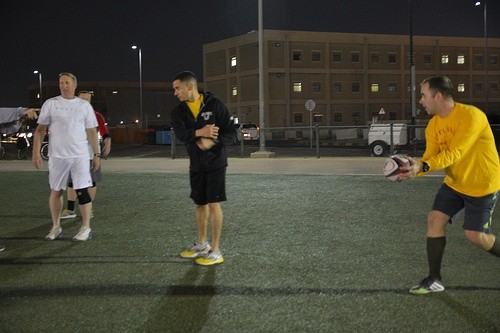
[367,122,426,157]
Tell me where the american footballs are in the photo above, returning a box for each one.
[383,155,411,183]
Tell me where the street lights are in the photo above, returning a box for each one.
[474,0,490,114]
[131,45,143,121]
[33,70,43,104]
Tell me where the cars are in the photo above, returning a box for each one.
[234,122,260,146]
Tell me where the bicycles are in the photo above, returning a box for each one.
[0,133,6,160]
[15,132,48,161]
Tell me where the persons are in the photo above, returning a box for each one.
[29,70,102,242]
[0,106,42,252]
[170,72,241,265]
[397,73,500,294]
[59,90,112,219]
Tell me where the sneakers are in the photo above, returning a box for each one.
[179,242,210,259]
[194,250,224,265]
[59,209,77,218]
[46,226,63,240]
[410,276,445,294]
[72,225,94,240]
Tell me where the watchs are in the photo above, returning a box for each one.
[421,161,430,172]
